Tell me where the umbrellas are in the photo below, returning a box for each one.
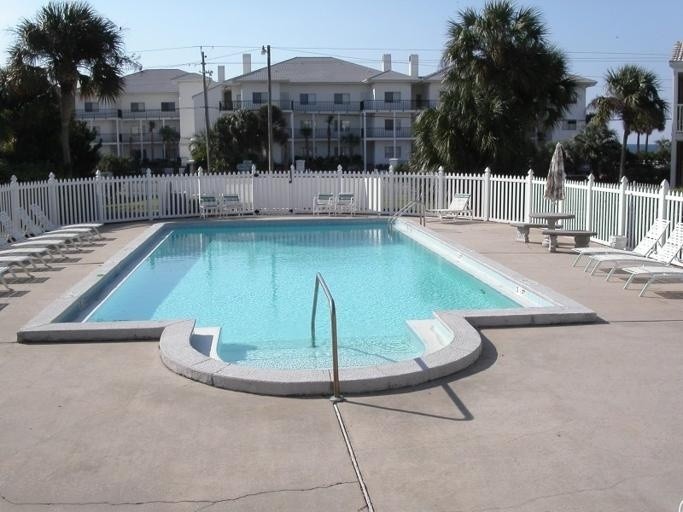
[541,141,566,201]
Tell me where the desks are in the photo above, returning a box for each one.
[529,212,575,247]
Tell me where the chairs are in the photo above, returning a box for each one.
[570,218,683,297]
[334,192,356,217]
[313,192,334,216]
[220,193,244,217]
[199,195,222,221]
[425,193,473,224]
[0,203,105,297]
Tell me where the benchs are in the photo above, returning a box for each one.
[542,230,597,252]
[510,222,563,243]
